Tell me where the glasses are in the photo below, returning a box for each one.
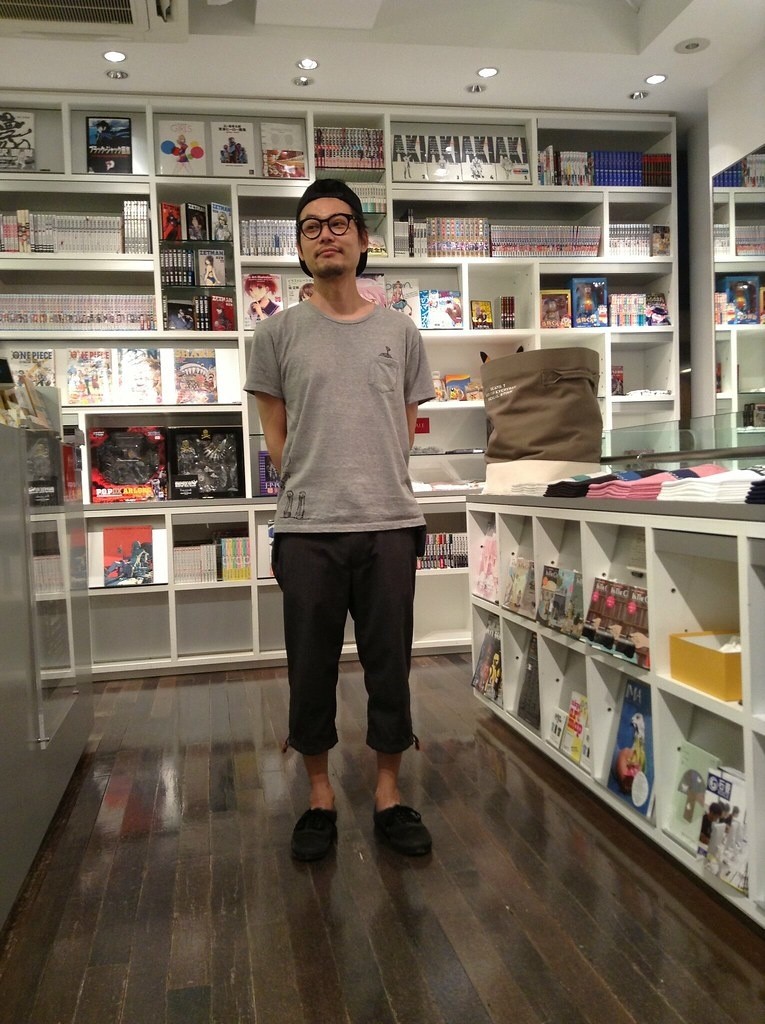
[297,213,357,239]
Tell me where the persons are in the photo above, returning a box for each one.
[244,180,437,861]
[699,801,739,845]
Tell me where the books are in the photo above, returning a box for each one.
[173,531,250,583]
[500,554,535,621]
[470,615,503,709]
[580,576,651,669]
[472,514,500,607]
[545,686,591,775]
[314,127,671,331]
[416,532,468,570]
[0,294,158,331]
[606,679,664,818]
[0,200,152,254]
[710,152,765,428]
[158,202,236,331]
[242,274,314,331]
[0,111,309,180]
[535,563,583,640]
[0,348,219,429]
[240,219,300,256]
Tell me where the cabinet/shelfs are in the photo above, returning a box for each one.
[712,153,765,467]
[0,90,681,683]
[466,502,765,931]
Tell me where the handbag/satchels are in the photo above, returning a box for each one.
[479,347,603,463]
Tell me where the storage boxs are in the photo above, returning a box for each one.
[167,425,245,500]
[564,278,609,327]
[717,275,759,325]
[540,289,571,328]
[670,629,741,701]
[88,426,168,504]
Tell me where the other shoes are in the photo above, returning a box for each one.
[291,808,338,861]
[373,805,432,856]
